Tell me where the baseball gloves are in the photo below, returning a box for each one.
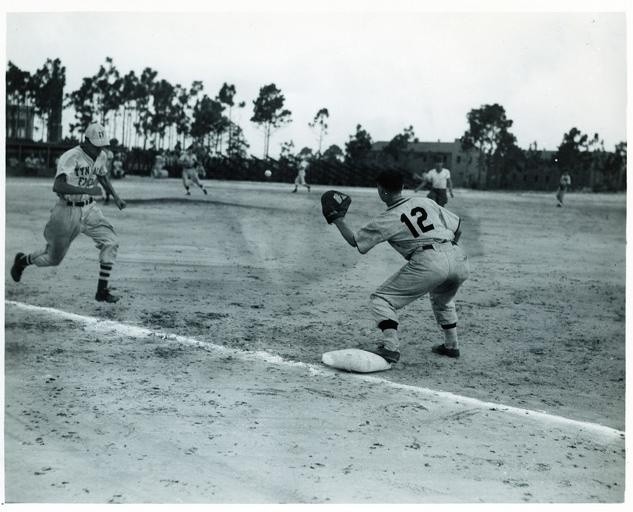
[319,191,351,223]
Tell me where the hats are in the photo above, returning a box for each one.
[85,123,111,147]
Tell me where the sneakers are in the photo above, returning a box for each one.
[374,345,400,363]
[95,289,118,302]
[432,343,459,357]
[11,252,29,282]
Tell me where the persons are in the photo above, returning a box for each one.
[290,154,312,194]
[319,168,471,365]
[150,151,169,179]
[411,153,454,209]
[8,122,129,302]
[175,146,208,196]
[554,169,572,209]
[7,145,129,207]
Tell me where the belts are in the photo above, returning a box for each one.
[67,197,92,206]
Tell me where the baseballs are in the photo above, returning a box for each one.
[261,170,273,177]
